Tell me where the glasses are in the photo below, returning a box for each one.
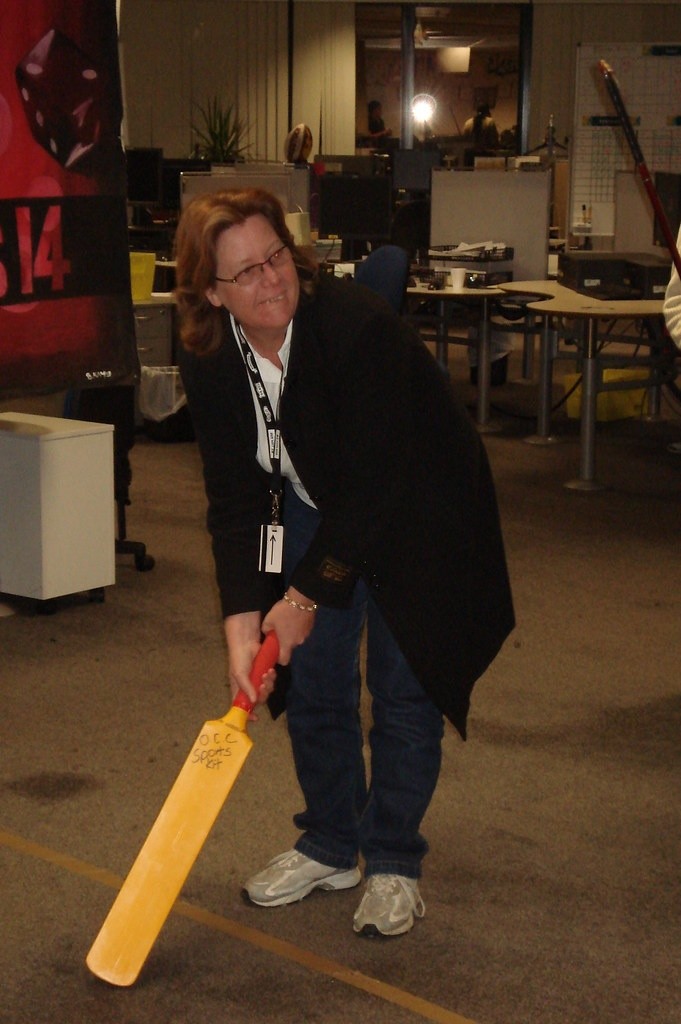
[211,243,291,288]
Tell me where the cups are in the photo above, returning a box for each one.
[451,268,466,289]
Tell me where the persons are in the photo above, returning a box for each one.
[368,99,393,147]
[461,101,498,152]
[662,222,681,349]
[174,184,516,939]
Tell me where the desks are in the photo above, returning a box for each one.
[405,266,511,370]
[487,280,665,492]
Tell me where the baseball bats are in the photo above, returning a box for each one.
[82,630,283,993]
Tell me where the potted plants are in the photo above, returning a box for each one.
[187,92,258,177]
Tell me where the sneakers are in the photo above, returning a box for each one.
[351,873,428,936]
[241,849,361,907]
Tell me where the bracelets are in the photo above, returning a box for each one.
[284,592,319,612]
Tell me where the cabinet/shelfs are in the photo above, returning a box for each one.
[133,306,172,368]
[0,411,116,616]
